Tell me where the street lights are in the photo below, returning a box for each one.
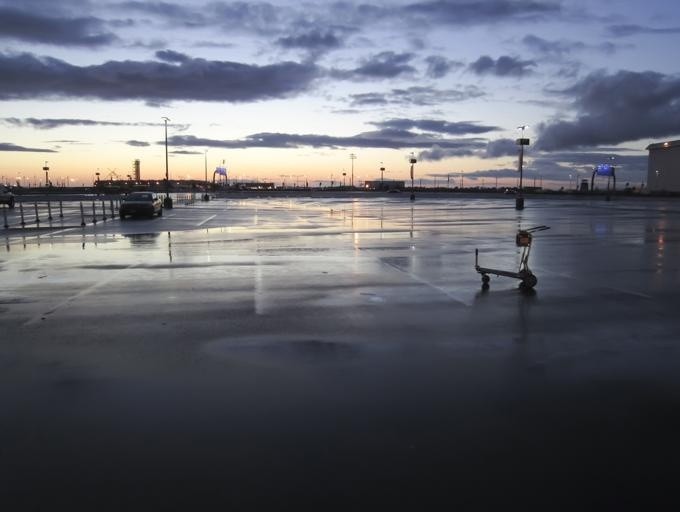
[516,124,530,199]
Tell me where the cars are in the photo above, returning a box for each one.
[0,183,16,208]
[119,192,162,221]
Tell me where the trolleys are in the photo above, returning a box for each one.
[475,225,550,290]
